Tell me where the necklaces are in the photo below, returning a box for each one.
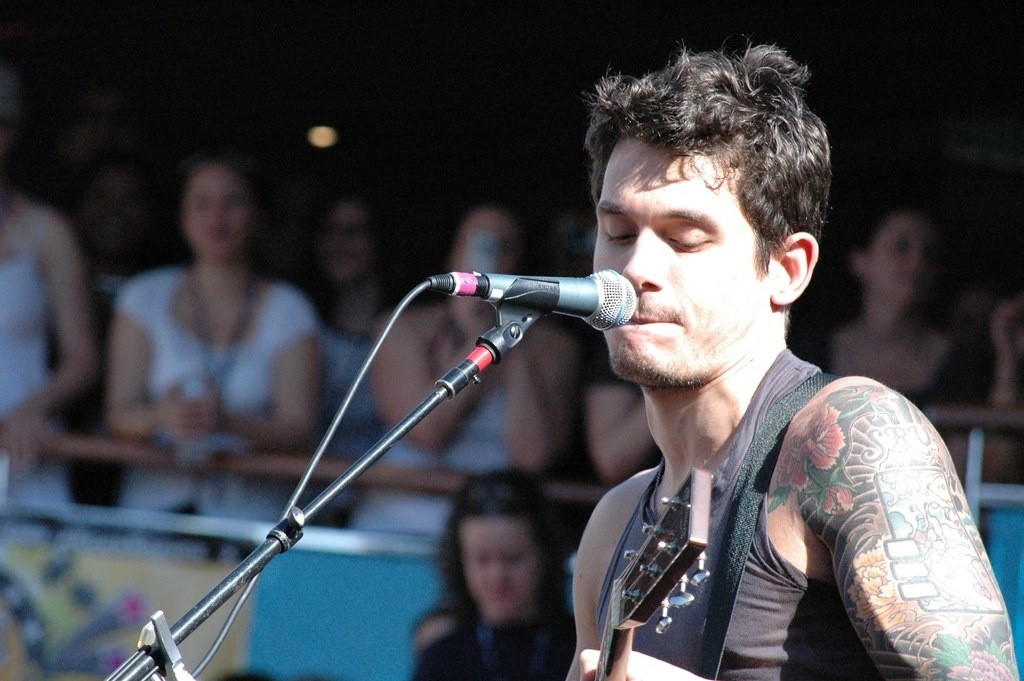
[653,452,665,513]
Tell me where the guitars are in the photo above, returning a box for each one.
[593,463,715,681]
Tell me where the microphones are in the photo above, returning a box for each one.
[426,268,637,331]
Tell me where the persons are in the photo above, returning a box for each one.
[409,465,577,681]
[0,64,1024,539]
[564,40,1023,681]
